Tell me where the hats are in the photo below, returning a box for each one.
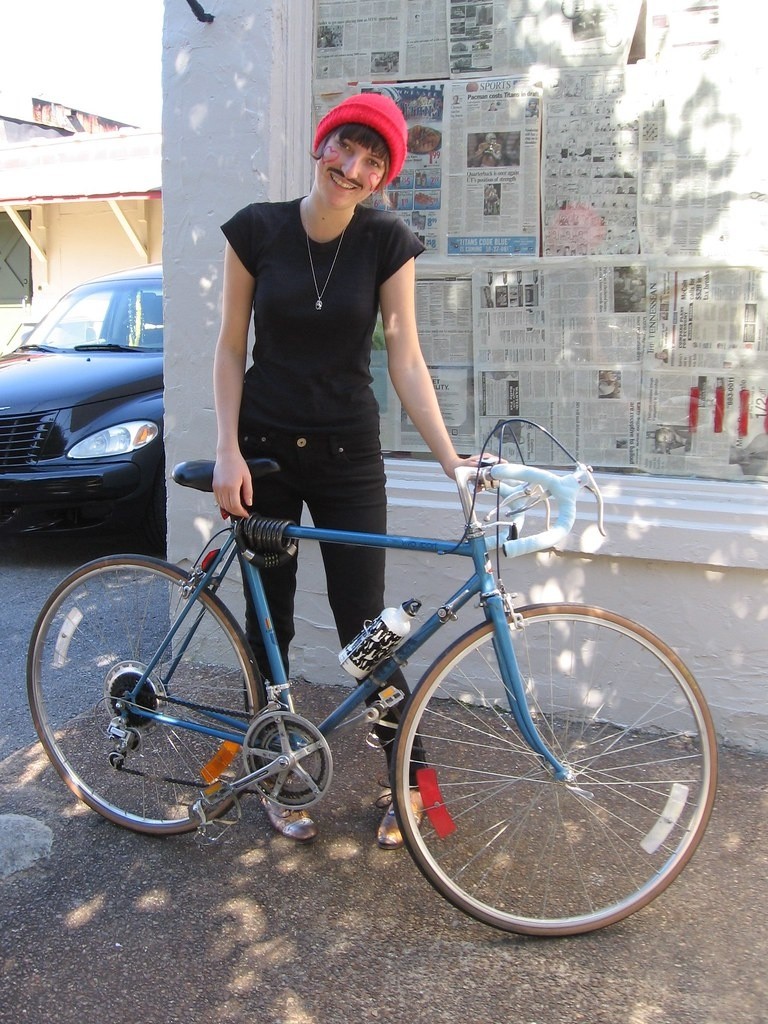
[313,93,407,191]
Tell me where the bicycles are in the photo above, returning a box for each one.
[23,416,720,940]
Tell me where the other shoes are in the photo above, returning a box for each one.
[261,795,319,846]
[376,780,424,849]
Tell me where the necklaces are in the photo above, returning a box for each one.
[306,197,352,311]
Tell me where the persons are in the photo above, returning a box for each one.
[525,99,539,117]
[655,425,691,446]
[453,95,459,104]
[212,93,497,849]
[475,133,501,168]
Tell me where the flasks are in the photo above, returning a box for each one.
[338,597,422,680]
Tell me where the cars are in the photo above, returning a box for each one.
[0,263,165,558]
